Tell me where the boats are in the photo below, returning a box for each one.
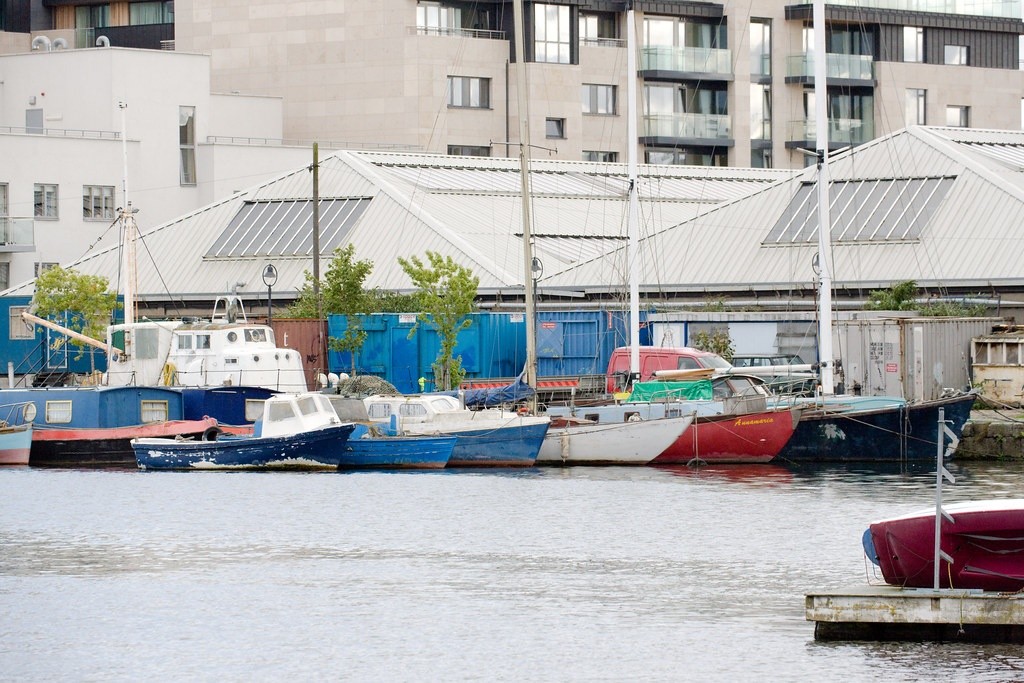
[0,0,988,471]
[862,496,1024,589]
[130,393,358,470]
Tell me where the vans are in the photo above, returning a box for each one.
[605,347,769,402]
[721,353,819,398]
